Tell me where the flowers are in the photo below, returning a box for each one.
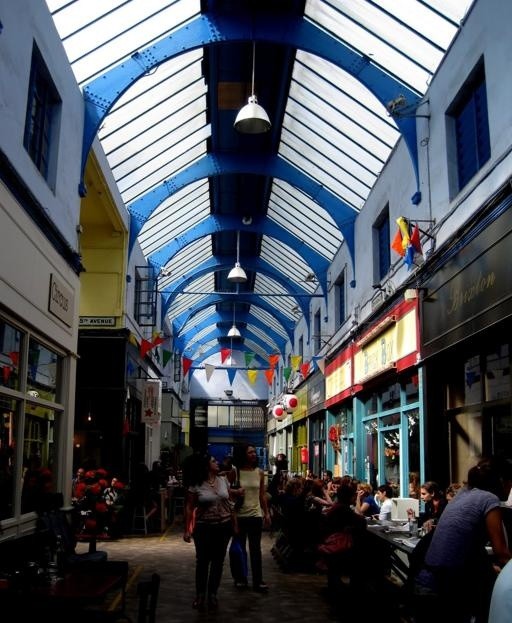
[76,470,129,551]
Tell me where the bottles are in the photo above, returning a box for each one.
[409,519,418,539]
[168,476,177,485]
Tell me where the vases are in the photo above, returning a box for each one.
[81,547,110,579]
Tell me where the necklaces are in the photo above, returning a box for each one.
[208,479,215,487]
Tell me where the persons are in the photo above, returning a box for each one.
[183,452,238,613]
[487,558,512,623]
[269,453,462,578]
[227,441,272,591]
[415,455,512,623]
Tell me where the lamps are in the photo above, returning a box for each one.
[270,388,306,422]
[230,42,272,138]
[221,231,249,369]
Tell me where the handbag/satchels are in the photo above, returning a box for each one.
[229,467,244,511]
[228,536,249,582]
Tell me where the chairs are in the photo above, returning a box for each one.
[268,478,511,623]
[117,463,185,539]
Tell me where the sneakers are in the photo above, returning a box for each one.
[207,594,219,612]
[235,580,248,589]
[253,579,270,590]
[191,592,205,610]
